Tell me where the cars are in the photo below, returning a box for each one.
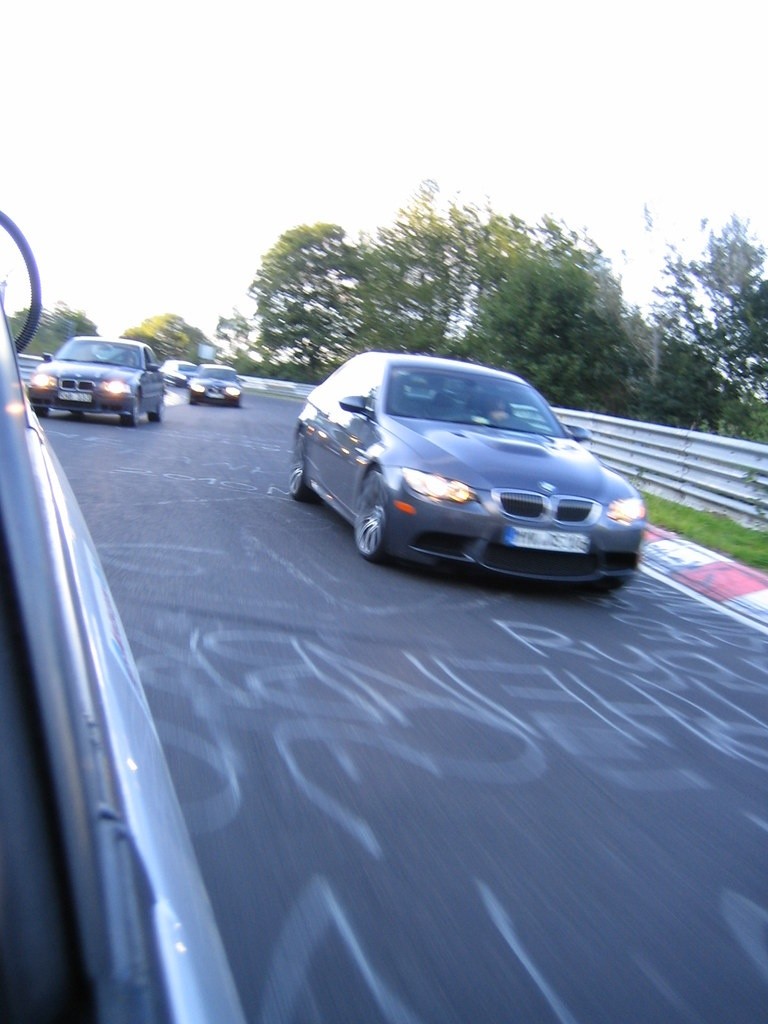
[25,334,165,428]
[187,362,243,406]
[284,349,648,591]
[157,359,200,387]
